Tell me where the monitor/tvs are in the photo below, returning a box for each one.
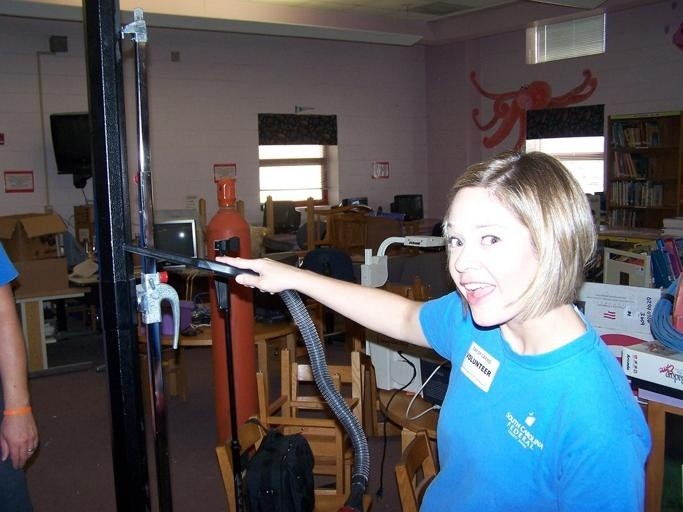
[154,219,198,258]
[390,194,423,221]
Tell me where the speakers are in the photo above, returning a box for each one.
[49,112,92,188]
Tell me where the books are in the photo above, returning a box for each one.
[608,120,683,237]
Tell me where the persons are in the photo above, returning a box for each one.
[0,242,41,512]
[215,149,653,511]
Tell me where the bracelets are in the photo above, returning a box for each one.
[2,406,33,417]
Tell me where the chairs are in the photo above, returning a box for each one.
[214,332,373,512]
[395,430,434,512]
[353,277,426,436]
[264,198,434,263]
[602,247,652,288]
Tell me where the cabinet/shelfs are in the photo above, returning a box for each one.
[605,110,683,230]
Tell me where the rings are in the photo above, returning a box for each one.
[26,447,34,452]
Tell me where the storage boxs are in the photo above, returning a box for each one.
[1,212,69,264]
[10,256,70,297]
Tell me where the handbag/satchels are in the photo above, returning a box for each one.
[245,428,316,512]
[305,247,354,285]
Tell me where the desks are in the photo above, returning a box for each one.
[15,287,95,379]
[135,303,296,417]
[594,223,683,247]
[634,398,683,512]
[379,389,434,491]
